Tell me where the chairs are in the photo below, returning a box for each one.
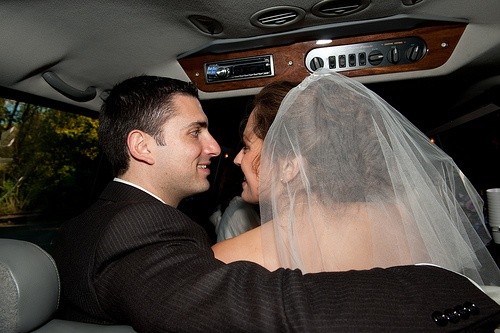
[0,238,139,333]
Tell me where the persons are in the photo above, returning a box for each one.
[210,80,414,273]
[47,75,500,333]
